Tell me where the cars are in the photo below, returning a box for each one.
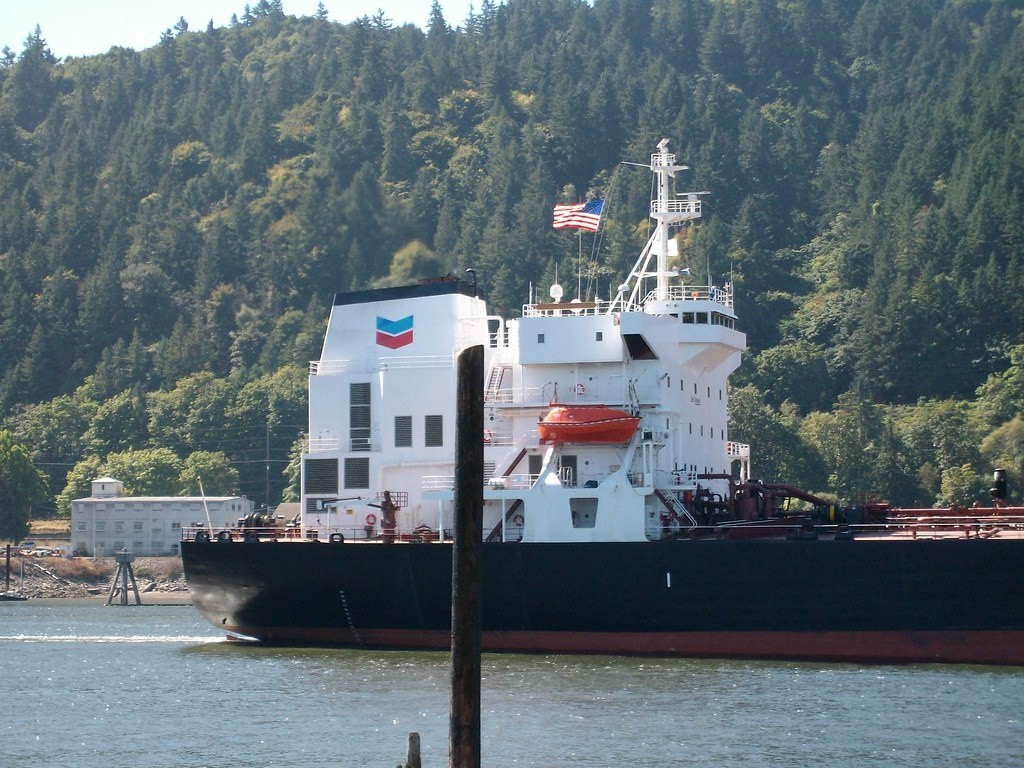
[0,541,88,558]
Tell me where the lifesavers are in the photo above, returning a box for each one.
[483,431,493,443]
[366,514,376,525]
[574,384,585,397]
[513,515,524,526]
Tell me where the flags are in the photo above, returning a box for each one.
[552,199,604,232]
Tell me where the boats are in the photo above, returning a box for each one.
[532,400,644,445]
[175,132,1024,668]
